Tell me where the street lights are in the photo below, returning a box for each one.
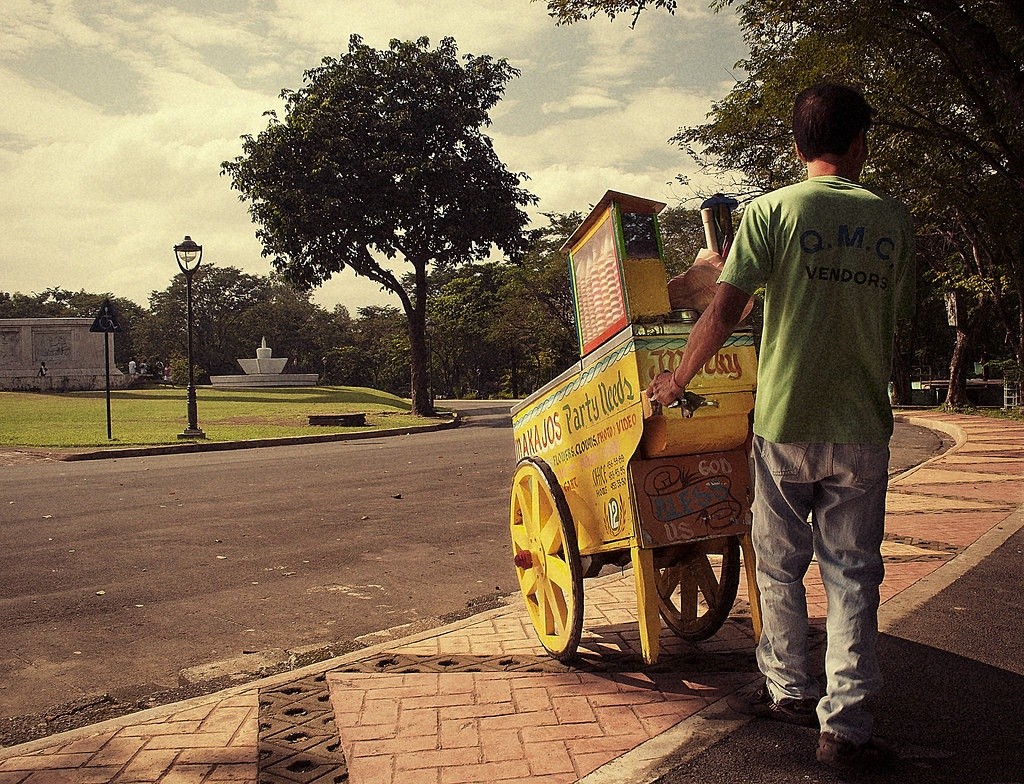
[174,235,209,441]
[425,318,436,408]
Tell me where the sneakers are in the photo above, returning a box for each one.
[816,731,896,768]
[725,681,820,728]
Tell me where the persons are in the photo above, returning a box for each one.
[129,358,174,388]
[646,84,917,769]
[38,362,46,377]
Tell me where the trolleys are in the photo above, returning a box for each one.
[509,190,761,667]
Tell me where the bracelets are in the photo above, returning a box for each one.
[673,366,689,389]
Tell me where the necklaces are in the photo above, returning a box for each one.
[816,173,845,177]
